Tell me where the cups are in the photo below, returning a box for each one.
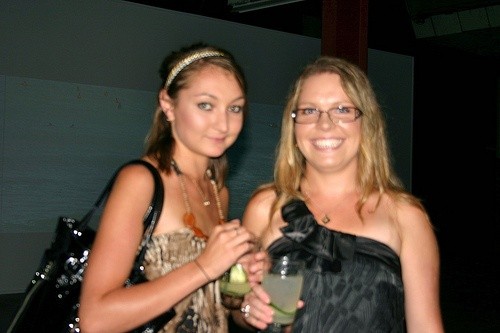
[220,264,249,309]
[260,258,305,333]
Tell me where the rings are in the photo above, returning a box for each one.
[241,304,250,313]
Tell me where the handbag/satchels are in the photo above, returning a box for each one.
[6,160,177,333]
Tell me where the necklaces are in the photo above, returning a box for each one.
[190,175,211,206]
[321,196,347,226]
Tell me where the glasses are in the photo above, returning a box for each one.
[292,107,363,125]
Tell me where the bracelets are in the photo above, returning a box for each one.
[193,261,211,281]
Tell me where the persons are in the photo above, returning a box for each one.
[229,58,444,333]
[79,45,266,333]
